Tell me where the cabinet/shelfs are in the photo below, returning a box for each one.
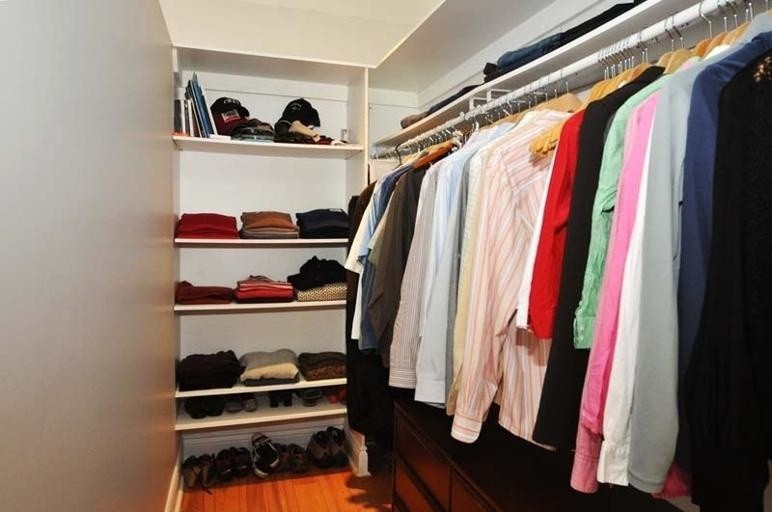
[363,1,667,144]
[388,396,540,512]
[174,44,362,434]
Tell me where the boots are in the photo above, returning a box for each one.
[307,431,331,467]
[328,427,348,467]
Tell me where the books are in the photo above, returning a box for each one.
[171,70,219,140]
[210,134,232,141]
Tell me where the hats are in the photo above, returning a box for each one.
[210,97,249,117]
[279,98,320,127]
[212,109,247,134]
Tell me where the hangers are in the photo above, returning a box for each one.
[367,63,585,182]
[529,1,769,159]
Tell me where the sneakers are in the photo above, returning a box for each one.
[268,390,280,407]
[252,433,280,468]
[184,398,206,418]
[252,448,269,478]
[225,395,241,411]
[217,450,232,481]
[325,386,347,403]
[297,387,321,406]
[289,444,308,473]
[233,447,250,477]
[240,393,256,412]
[182,456,199,487]
[199,454,214,488]
[206,396,225,415]
[283,390,291,406]
[274,444,288,472]
